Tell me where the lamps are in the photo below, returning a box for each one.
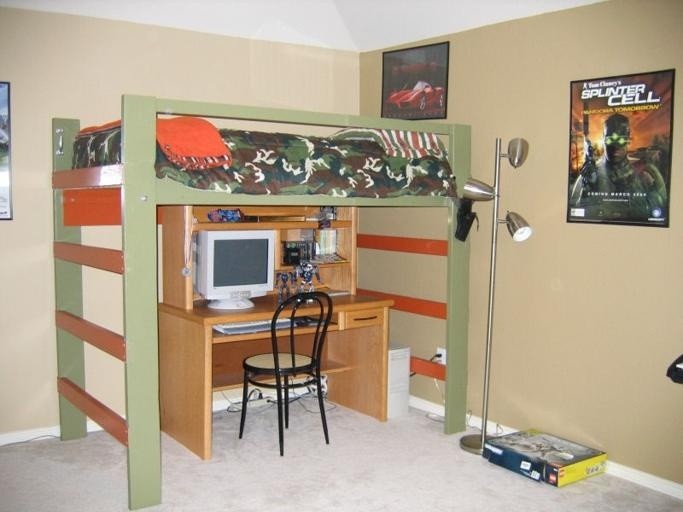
[459,136,533,458]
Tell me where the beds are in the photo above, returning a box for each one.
[50,91,474,511]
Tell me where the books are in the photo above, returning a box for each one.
[314,286,352,297]
[281,226,350,267]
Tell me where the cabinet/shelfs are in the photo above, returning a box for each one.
[160,203,358,312]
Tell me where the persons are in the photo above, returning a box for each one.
[571,111,669,224]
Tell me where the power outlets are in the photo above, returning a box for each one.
[435,347,447,365]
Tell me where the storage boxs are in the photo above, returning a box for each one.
[483,427,609,489]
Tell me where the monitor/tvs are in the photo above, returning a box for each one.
[197,228,275,310]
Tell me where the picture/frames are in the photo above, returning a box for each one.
[0,81,14,220]
[566,67,676,228]
[380,40,450,120]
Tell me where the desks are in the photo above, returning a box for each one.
[156,285,396,461]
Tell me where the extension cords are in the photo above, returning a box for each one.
[230,397,273,413]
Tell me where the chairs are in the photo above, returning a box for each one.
[239,291,334,457]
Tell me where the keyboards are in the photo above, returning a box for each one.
[214,319,296,335]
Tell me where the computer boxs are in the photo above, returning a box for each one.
[388,344,410,420]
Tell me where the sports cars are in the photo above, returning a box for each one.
[384,78,445,113]
